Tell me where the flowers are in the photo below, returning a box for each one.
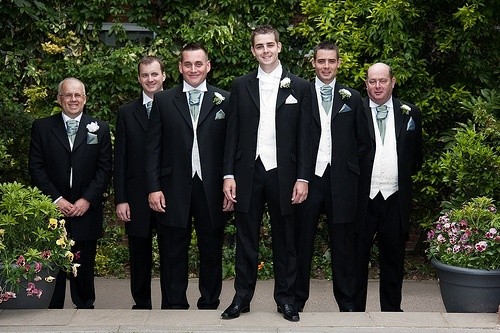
[0,181,81,303]
[280,77,290,89]
[213,92,225,105]
[338,88,352,100]
[422,195,500,272]
[399,102,411,115]
[86,121,100,133]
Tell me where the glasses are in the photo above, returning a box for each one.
[60,94,84,100]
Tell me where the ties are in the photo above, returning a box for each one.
[377,105,388,144]
[147,101,153,118]
[67,120,78,152]
[320,85,332,114]
[261,75,274,107]
[189,90,200,121]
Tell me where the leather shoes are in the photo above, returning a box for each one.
[221,302,250,319]
[277,303,299,321]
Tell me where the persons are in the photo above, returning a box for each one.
[142,42,231,311]
[221,23,315,321]
[115,55,189,310]
[28,77,112,309]
[292,40,373,313]
[348,61,423,312]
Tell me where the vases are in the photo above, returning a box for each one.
[0,265,57,310]
[431,258,500,314]
